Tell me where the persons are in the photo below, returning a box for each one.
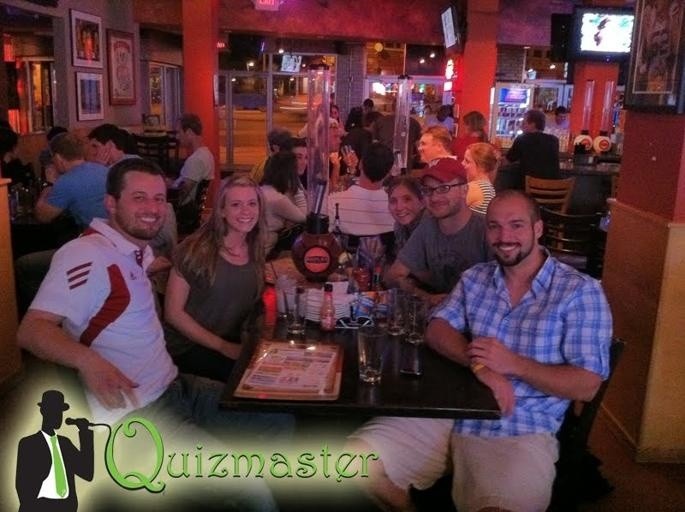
[2,91,614,511]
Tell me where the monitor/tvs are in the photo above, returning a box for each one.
[572,4,635,62]
[440,5,461,49]
[551,13,572,62]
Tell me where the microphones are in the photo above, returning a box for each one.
[65,418,93,426]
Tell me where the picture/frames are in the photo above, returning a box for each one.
[104,28,136,107]
[621,2,685,114]
[73,72,105,123]
[68,9,104,69]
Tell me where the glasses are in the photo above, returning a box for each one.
[419,182,466,197]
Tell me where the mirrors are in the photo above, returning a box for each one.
[3,4,62,138]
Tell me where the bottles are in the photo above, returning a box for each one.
[593,128,612,153]
[574,128,593,153]
[21,173,34,216]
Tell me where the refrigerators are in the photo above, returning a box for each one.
[491,81,574,150]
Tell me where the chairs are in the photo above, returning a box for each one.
[0,127,628,512]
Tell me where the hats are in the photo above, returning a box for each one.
[419,157,468,184]
[38,390,70,411]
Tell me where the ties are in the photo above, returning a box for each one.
[51,435,67,497]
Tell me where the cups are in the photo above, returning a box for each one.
[281,288,308,334]
[341,145,353,163]
[292,213,430,383]
[274,269,296,317]
